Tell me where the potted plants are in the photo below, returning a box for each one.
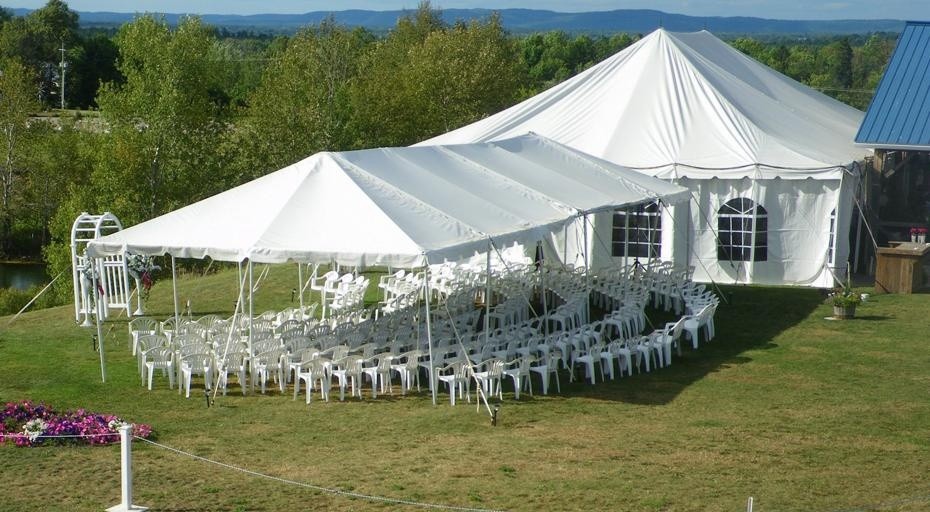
[828,290,870,320]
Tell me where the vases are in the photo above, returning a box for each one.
[911,234,925,245]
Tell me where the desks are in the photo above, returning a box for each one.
[872,239,929,295]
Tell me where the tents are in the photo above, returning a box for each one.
[405,27,897,291]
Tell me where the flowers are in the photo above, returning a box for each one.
[80,265,105,317]
[0,399,154,448]
[910,227,928,233]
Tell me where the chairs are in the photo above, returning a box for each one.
[127,255,721,415]
